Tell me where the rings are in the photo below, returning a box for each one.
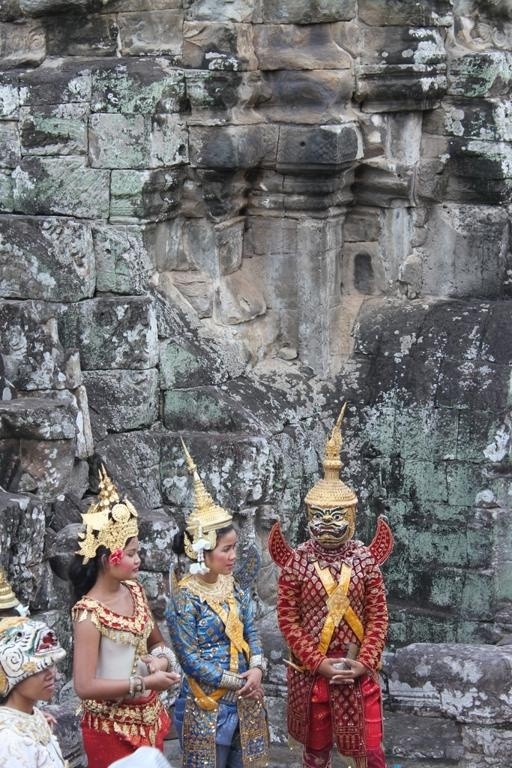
[248,687,253,692]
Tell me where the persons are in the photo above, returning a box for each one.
[165,434,269,768]
[0,615,67,768]
[72,460,182,768]
[0,570,58,727]
[267,400,394,768]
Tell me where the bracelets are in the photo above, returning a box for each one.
[129,675,144,697]
[152,646,176,672]
